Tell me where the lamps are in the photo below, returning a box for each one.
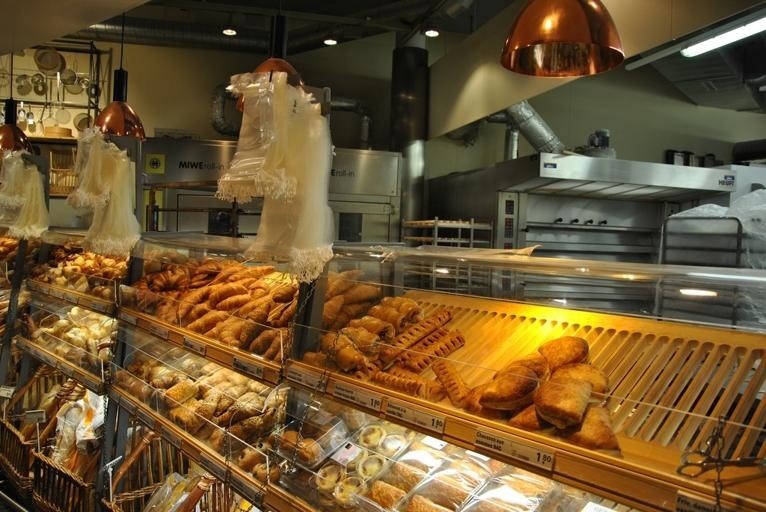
[499,0,624,78]
[0,54,32,155]
[91,13,146,141]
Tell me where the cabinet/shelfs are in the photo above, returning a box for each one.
[0,234,764,512]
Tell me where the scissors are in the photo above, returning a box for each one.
[677,450,766,478]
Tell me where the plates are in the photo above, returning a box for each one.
[575,145,617,160]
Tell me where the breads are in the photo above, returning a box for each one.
[30,307,120,380]
[41,390,108,511]
[113,341,554,511]
[301,296,621,451]
[124,254,379,365]
[31,251,163,303]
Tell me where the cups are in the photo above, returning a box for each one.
[589,129,610,148]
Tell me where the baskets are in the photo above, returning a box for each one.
[0,364,234,512]
